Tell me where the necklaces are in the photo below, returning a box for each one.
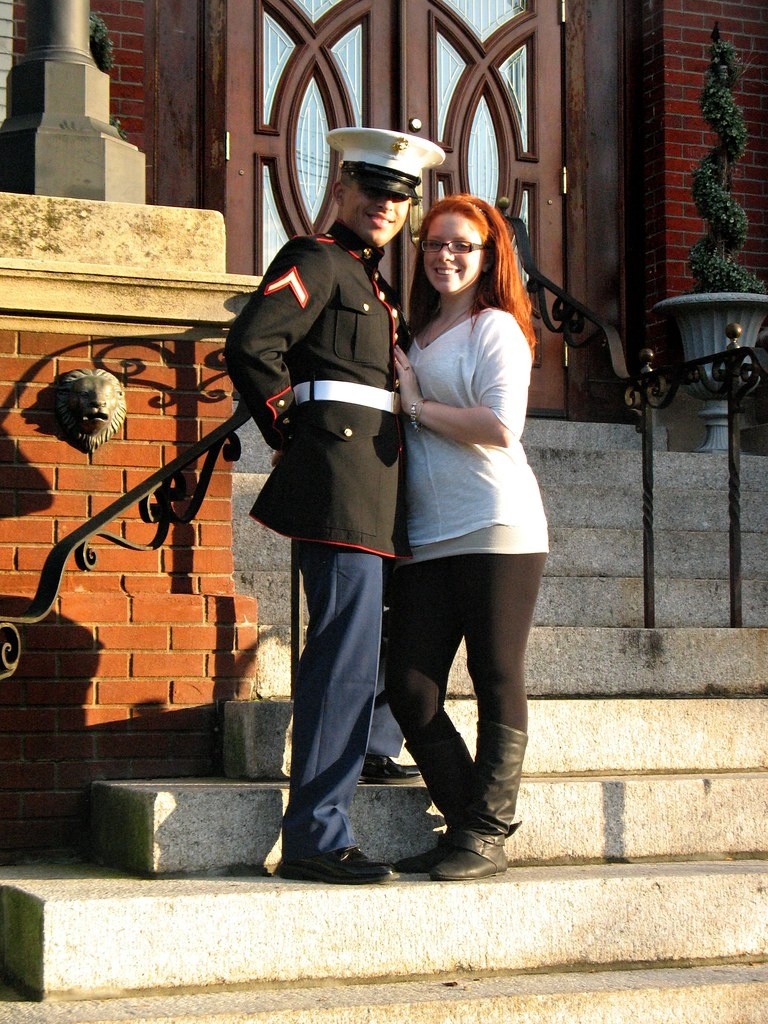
[421,309,467,350]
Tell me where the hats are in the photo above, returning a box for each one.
[325,127,446,198]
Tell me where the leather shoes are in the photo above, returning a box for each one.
[278,845,393,885]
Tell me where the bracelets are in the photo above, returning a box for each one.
[410,398,427,433]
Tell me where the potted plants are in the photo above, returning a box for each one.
[656,18,768,453]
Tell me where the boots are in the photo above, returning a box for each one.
[429,721,528,880]
[394,732,485,873]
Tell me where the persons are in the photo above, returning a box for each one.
[222,126,550,885]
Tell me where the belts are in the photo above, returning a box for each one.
[293,380,403,415]
[359,753,423,783]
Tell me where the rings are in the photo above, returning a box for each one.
[404,366,410,370]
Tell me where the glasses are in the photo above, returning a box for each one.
[420,240,489,253]
